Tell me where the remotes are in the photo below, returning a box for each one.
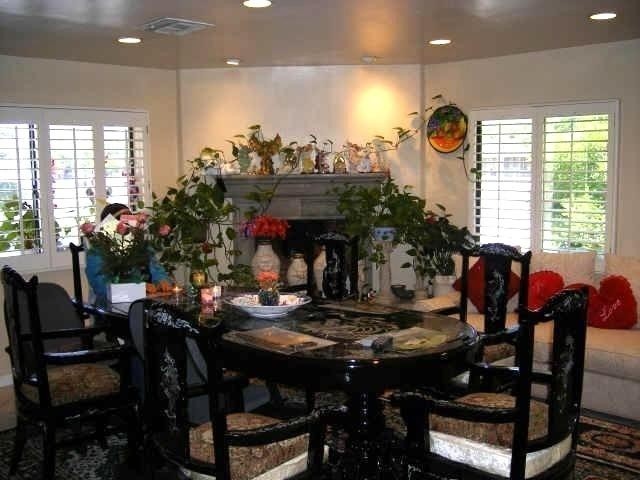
[372,335,394,349]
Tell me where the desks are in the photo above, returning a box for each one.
[92,283,479,477]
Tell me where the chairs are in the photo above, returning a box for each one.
[456,241,534,383]
[69,241,92,326]
[134,293,340,480]
[390,280,584,478]
[0,265,129,479]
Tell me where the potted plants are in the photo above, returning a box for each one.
[323,182,480,298]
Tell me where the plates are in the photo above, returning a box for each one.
[222,293,314,321]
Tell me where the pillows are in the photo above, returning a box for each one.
[564,274,639,330]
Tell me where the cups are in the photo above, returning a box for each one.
[172,271,222,304]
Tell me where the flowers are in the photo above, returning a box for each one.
[239,220,292,241]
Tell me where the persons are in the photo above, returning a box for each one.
[83,202,181,394]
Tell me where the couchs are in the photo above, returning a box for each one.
[413,248,640,424]
